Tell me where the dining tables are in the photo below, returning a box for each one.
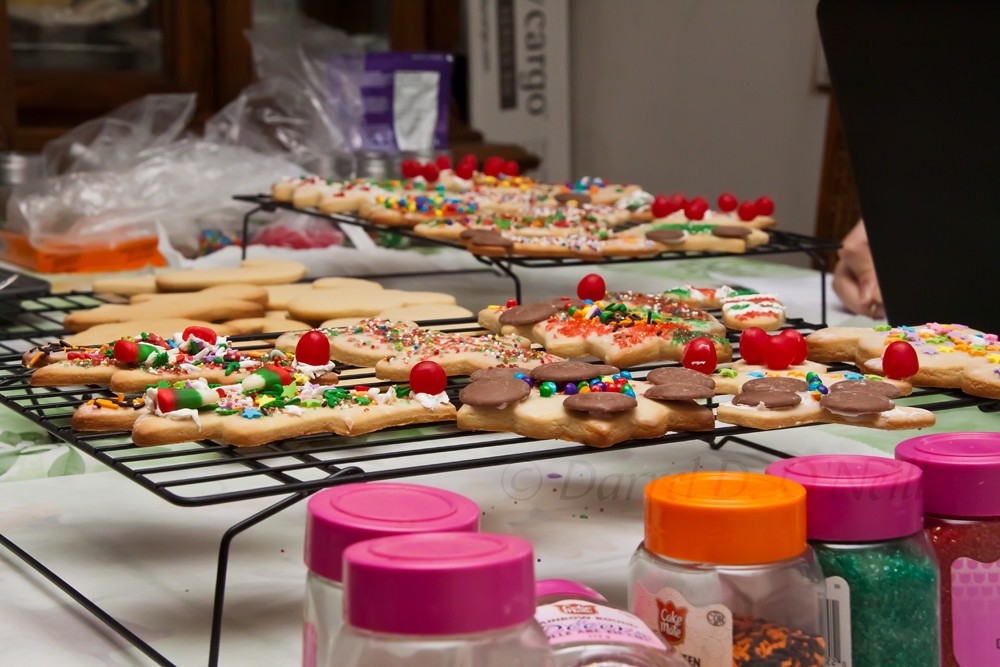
[0,247,1000,667]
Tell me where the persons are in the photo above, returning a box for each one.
[831,218,886,319]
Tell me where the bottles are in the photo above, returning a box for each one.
[765,454,943,667]
[531,577,693,667]
[299,481,481,667]
[893,431,1000,667]
[626,470,836,667]
[325,531,558,667]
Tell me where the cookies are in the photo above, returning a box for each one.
[270,152,775,256]
[23,253,1000,448]
